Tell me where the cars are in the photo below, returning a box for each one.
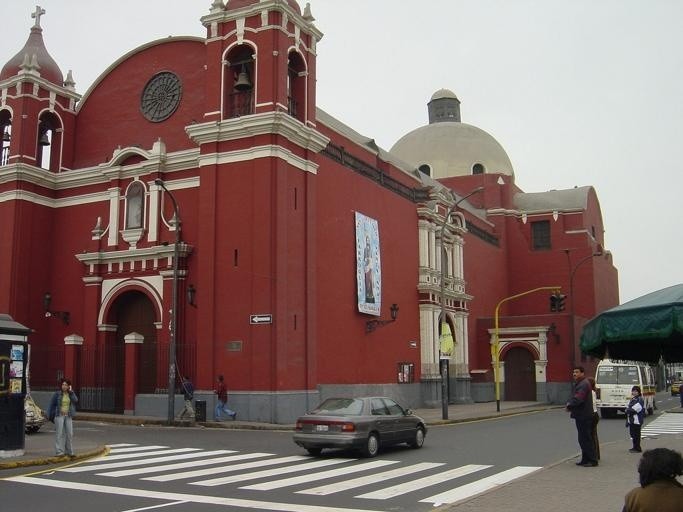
[292,394,429,459]
[670,380,683,395]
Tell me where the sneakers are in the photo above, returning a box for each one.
[629,448,642,453]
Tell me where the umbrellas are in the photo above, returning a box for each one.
[580,284,683,365]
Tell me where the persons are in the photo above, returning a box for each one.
[213,374,236,421]
[621,448,683,512]
[566,367,600,467]
[665,375,683,407]
[175,375,196,420]
[47,378,79,457]
[624,385,645,453]
[363,236,375,303]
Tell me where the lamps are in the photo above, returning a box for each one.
[366,304,399,334]
[186,284,197,306]
[44,292,69,326]
[549,323,560,343]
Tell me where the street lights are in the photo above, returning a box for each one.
[436,183,485,419]
[148,176,184,421]
[562,244,603,407]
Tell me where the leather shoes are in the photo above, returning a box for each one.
[576,459,598,466]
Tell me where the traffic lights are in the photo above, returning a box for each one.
[549,294,557,312]
[558,292,568,311]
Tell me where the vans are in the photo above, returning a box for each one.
[592,362,658,418]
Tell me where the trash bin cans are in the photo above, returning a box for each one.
[195,400,206,422]
